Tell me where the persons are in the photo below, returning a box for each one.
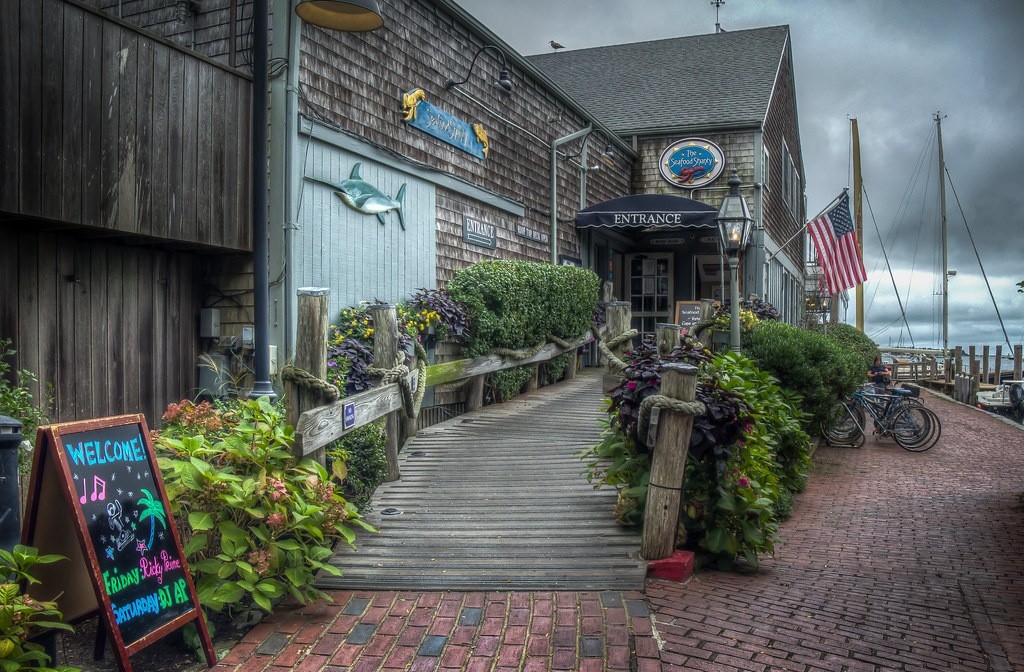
[867,357,891,411]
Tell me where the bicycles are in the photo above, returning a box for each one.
[818,382,941,453]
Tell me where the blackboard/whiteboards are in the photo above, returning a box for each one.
[49,411,202,653]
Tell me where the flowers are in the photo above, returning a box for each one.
[149,397,378,619]
[587,337,790,555]
[331,303,441,398]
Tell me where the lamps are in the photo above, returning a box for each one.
[294,0,384,33]
[563,129,615,168]
[444,45,512,93]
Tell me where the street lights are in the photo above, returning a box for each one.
[714,168,756,358]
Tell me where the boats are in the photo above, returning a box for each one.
[976,379,1024,412]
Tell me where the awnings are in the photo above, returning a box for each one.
[576,194,753,257]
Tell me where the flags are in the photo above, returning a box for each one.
[807,191,867,296]
